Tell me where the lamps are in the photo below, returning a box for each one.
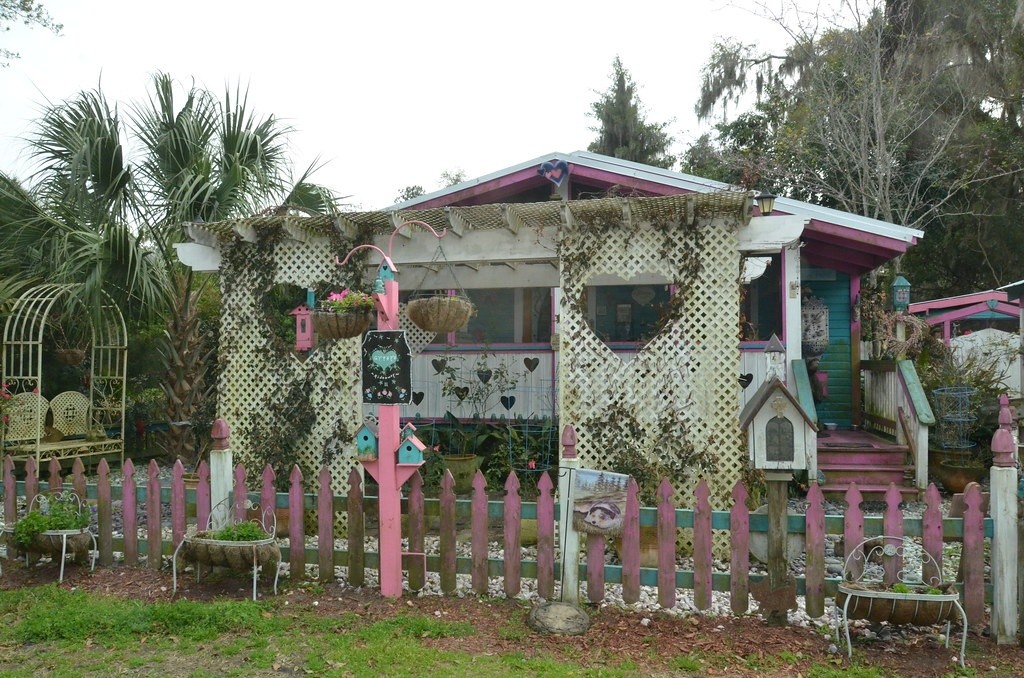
[755,188,778,216]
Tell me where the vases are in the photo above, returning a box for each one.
[311,308,374,338]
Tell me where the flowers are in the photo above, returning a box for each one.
[0,383,39,424]
[310,288,374,313]
[876,312,924,355]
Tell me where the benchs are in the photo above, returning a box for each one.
[0,282,128,482]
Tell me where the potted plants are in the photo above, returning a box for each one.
[0,487,97,584]
[432,339,530,496]
[915,337,1009,494]
[575,393,719,569]
[132,388,168,433]
[836,536,968,671]
[231,371,319,538]
[182,394,216,516]
[171,493,281,603]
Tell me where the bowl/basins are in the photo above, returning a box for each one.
[824,423,838,431]
